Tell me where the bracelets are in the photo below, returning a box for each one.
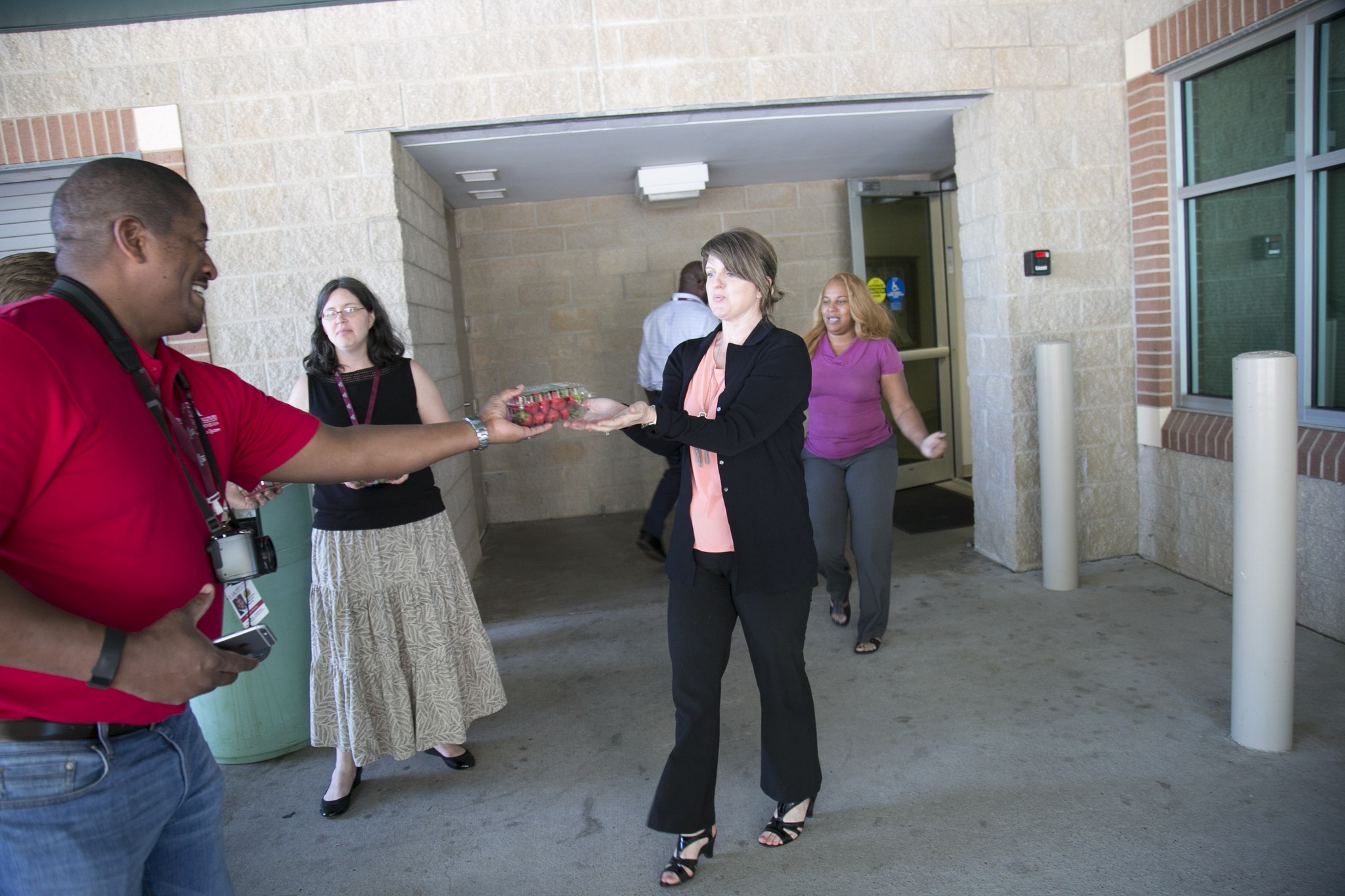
[462,416,489,453]
[84,623,126,688]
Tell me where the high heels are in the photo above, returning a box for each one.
[759,790,818,847]
[660,825,718,887]
[320,765,362,817]
[425,741,474,769]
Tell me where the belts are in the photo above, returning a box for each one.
[0,711,158,741]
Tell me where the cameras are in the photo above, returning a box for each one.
[206,509,276,585]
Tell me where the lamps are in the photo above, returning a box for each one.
[635,163,710,204]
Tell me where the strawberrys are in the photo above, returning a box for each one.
[507,387,584,427]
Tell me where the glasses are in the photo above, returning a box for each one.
[321,306,367,320]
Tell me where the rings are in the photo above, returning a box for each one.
[604,432,610,436]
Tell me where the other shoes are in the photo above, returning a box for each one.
[636,529,667,561]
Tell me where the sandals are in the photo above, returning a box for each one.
[829,595,851,627]
[854,637,882,654]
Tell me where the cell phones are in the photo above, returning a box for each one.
[210,624,277,656]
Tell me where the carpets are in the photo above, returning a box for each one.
[893,486,975,534]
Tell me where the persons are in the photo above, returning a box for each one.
[0,157,554,896]
[800,271,947,655]
[633,261,722,563]
[562,226,823,891]
[284,276,507,819]
[234,597,249,614]
